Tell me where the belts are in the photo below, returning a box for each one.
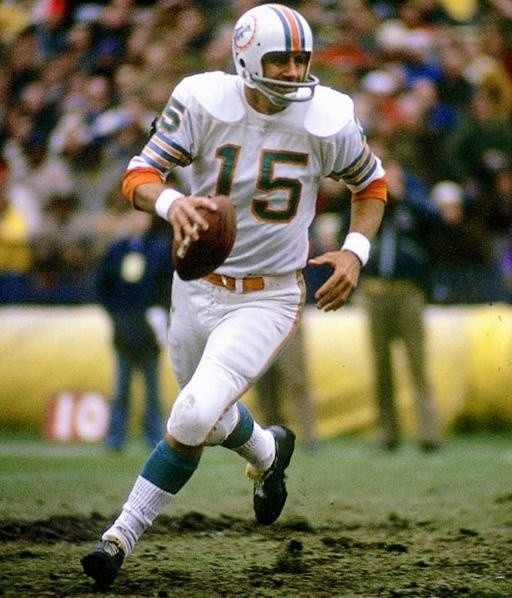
[203,272,302,291]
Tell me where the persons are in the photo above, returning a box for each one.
[102,252,169,451]
[364,160,440,452]
[79,5,389,584]
[2,1,512,300]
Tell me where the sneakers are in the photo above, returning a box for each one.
[80,540,124,585]
[246,424,296,524]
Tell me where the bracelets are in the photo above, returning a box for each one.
[154,189,185,224]
[340,232,371,266]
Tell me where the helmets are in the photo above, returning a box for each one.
[232,3,320,108]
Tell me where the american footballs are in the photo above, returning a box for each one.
[172,193,234,281]
